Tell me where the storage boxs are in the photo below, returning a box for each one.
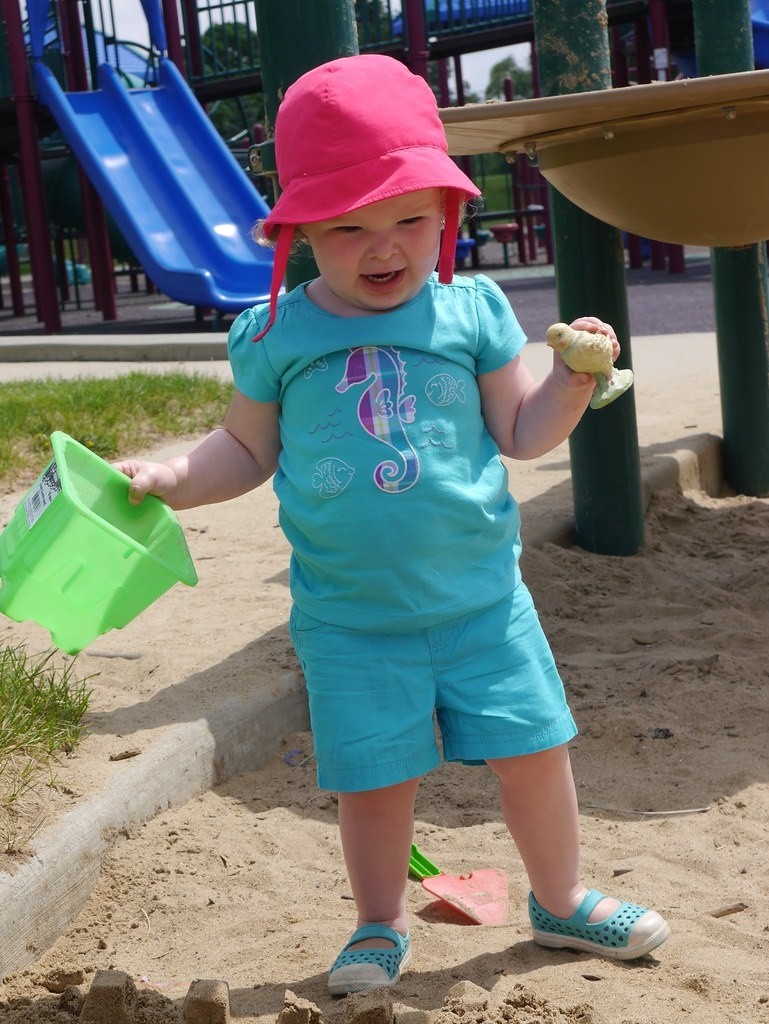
[1,430,198,658]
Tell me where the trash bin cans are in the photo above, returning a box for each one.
[285,240,321,295]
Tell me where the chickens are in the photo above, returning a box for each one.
[546,323,615,379]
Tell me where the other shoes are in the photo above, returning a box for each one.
[327,923,411,994]
[529,890,670,959]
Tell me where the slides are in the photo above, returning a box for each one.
[24,53,314,313]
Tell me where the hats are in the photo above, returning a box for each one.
[254,54,482,338]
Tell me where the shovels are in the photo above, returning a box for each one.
[409,844,510,926]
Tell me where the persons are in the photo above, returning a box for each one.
[101,50,671,994]
